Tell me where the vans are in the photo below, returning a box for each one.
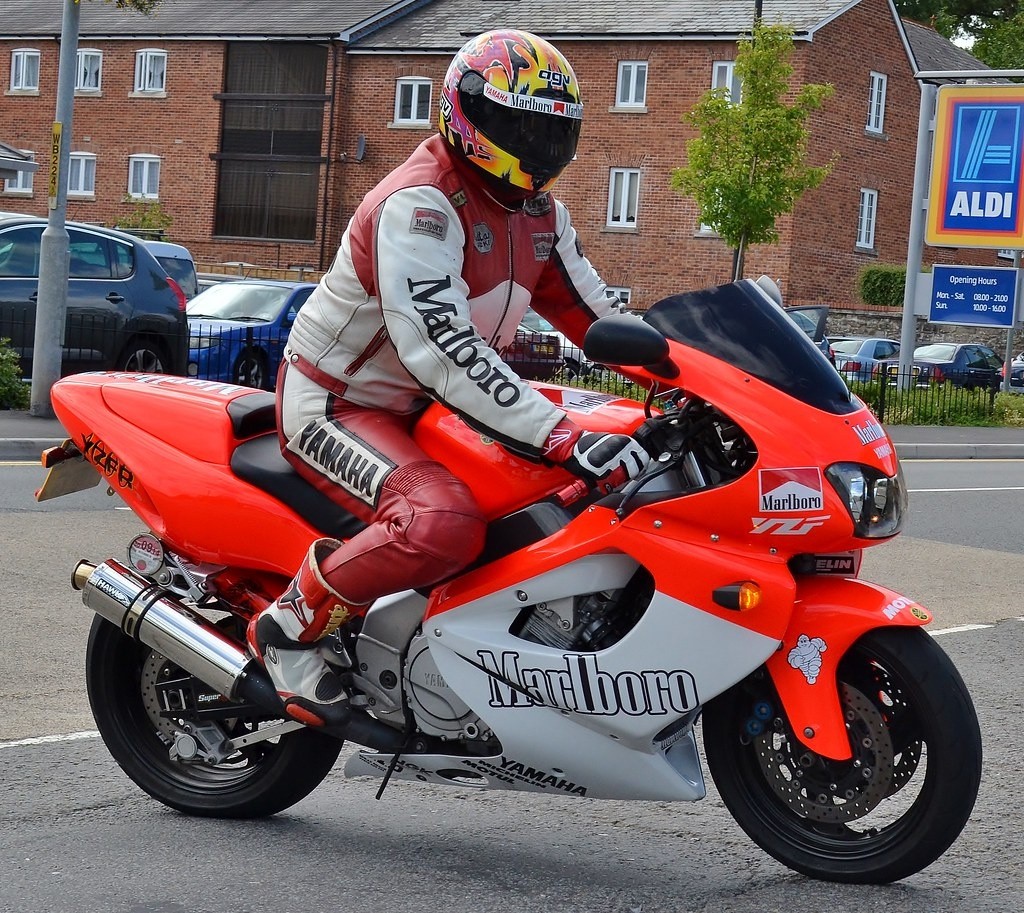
[70,240,198,300]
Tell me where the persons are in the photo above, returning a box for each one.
[247,29,695,726]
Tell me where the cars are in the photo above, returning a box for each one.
[826,336,902,381]
[1000,351,1024,392]
[501,322,566,380]
[186,281,319,393]
[872,341,1005,390]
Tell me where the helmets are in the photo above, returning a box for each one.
[438,29,582,193]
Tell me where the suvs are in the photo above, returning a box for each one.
[0,217,189,378]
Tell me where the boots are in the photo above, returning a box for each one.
[247,537,374,729]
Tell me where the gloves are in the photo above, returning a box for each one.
[542,416,650,494]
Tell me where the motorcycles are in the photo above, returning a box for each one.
[34,275,984,886]
